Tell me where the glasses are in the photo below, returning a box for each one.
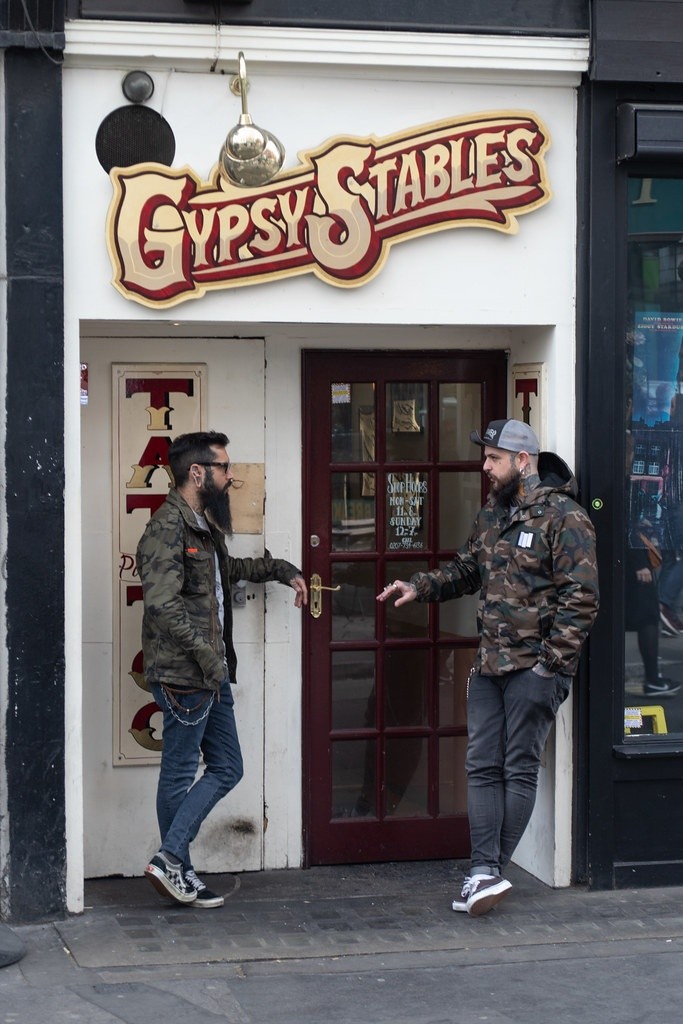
[188,462,232,475]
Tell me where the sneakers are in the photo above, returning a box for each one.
[643,678,681,696]
[658,610,683,637]
[452,874,512,917]
[145,852,224,908]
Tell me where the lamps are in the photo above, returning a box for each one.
[219,51,285,188]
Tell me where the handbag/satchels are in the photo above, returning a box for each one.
[639,532,661,569]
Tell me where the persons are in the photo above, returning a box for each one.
[620,380,683,698]
[135,432,308,908]
[377,420,600,920]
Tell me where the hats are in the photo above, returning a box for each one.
[469,417,540,456]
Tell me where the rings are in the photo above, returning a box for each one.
[393,584,398,589]
